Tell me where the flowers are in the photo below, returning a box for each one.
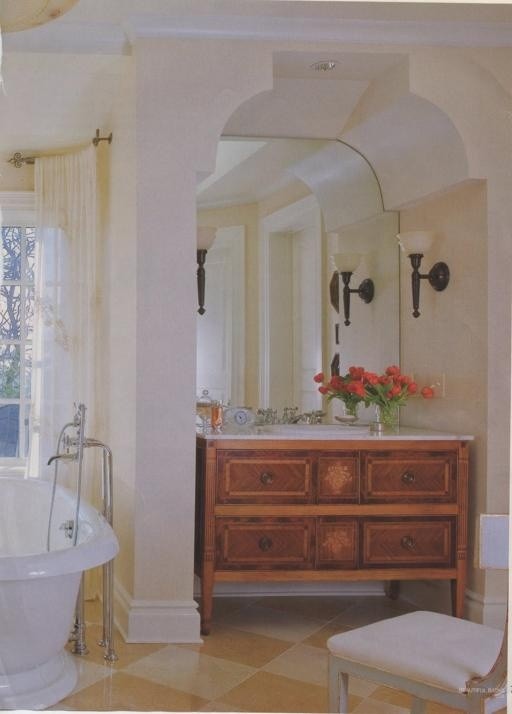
[348,365,435,423]
[313,371,374,416]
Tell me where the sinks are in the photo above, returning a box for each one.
[256,424,369,440]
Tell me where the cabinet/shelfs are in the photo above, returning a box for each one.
[201,440,469,636]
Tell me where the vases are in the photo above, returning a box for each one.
[342,401,360,418]
[376,404,400,432]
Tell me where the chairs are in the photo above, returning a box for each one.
[325,512,509,713]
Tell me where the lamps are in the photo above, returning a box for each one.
[329,252,374,326]
[395,230,451,319]
[197,226,218,316]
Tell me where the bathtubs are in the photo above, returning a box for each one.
[0,475,121,676]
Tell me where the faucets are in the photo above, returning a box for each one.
[256,408,279,425]
[46,446,79,467]
[283,407,310,425]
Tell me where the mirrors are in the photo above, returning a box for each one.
[197,134,402,426]
